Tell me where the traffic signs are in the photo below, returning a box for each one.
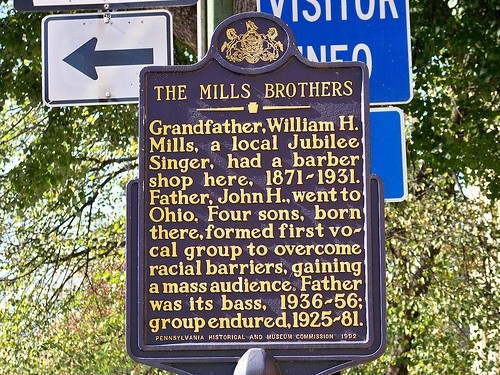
[252,0,412,106]
